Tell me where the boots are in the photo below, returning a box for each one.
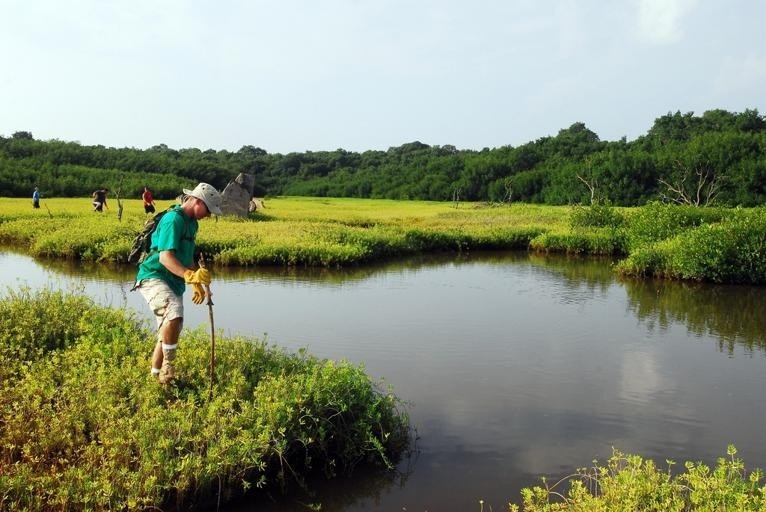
[151,343,185,388]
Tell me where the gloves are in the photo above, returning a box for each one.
[191,283,205,305]
[183,268,210,285]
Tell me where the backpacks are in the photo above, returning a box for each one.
[127,204,185,266]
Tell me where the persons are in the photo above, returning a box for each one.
[91,188,110,212]
[133,181,223,384]
[32,186,40,208]
[142,186,156,213]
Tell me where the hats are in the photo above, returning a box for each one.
[182,182,223,216]
